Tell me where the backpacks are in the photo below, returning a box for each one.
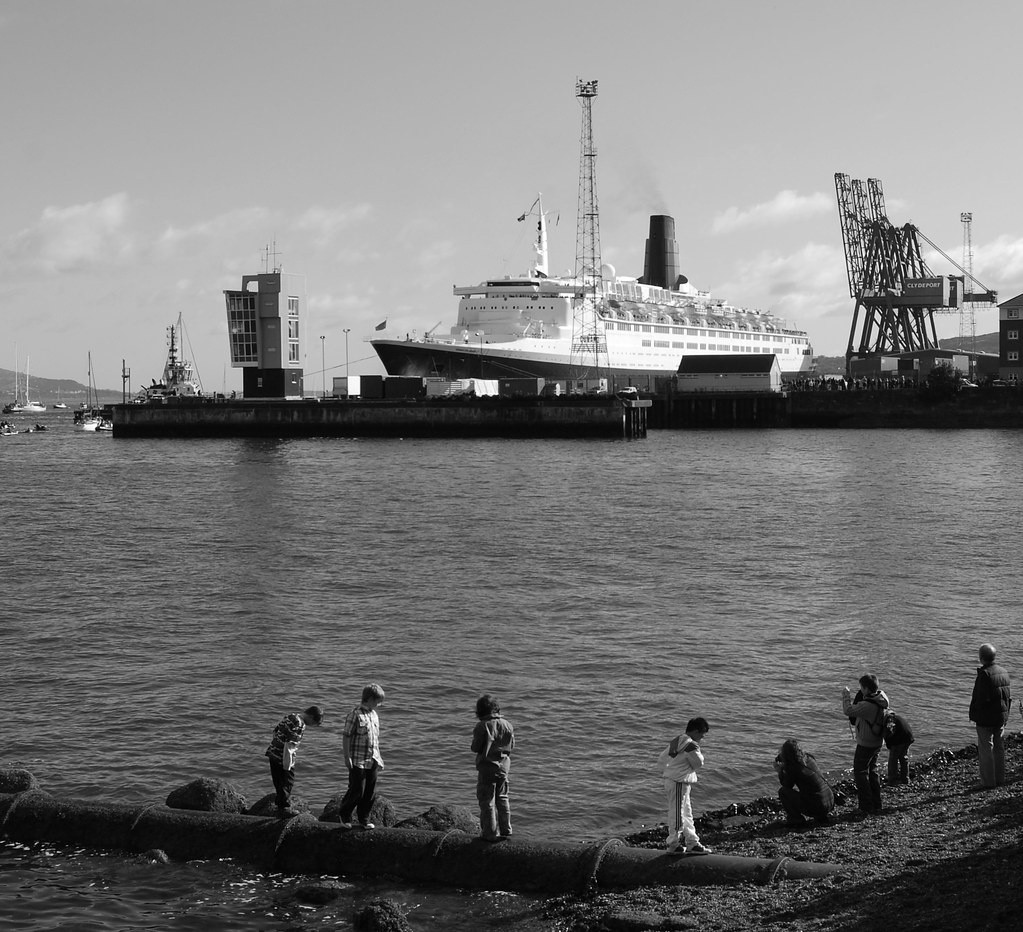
[862,702,895,738]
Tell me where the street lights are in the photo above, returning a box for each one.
[342,329,351,375]
[320,335,326,397]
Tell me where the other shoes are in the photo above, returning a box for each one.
[673,846,685,853]
[359,822,374,829]
[691,844,711,854]
[276,810,300,819]
[338,816,352,829]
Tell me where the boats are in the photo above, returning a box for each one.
[367,213,815,391]
[0,420,50,436]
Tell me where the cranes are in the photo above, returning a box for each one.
[833,171,998,384]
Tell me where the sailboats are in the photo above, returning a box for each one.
[2,340,47,414]
[53,384,68,408]
[72,350,113,433]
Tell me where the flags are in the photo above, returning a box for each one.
[518,214,525,222]
[375,321,386,331]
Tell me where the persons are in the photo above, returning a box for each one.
[471,695,515,839]
[782,374,919,390]
[339,683,385,829]
[773,739,835,831]
[657,717,712,855]
[969,644,1011,789]
[265,706,323,809]
[883,709,915,785]
[843,673,889,811]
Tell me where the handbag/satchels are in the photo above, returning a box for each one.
[832,789,847,806]
